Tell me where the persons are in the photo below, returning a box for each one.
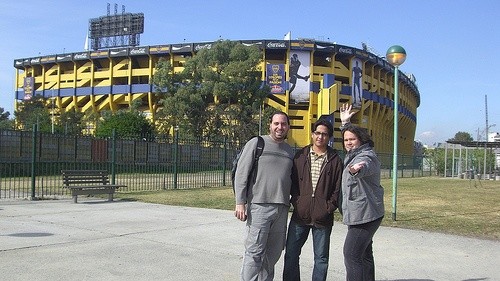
[234,110,295,281]
[339,102,385,281]
[352,61,363,104]
[283,119,343,281]
[290,54,310,94]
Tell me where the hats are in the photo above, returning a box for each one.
[312,115,333,133]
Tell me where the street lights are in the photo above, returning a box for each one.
[476,124,496,149]
[385,44,406,220]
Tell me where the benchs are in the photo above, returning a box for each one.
[60,170,128,203]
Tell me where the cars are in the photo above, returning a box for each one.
[488,170,500,179]
[460,169,482,179]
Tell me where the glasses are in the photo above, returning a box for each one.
[313,131,330,136]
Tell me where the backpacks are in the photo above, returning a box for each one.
[231,135,264,197]
